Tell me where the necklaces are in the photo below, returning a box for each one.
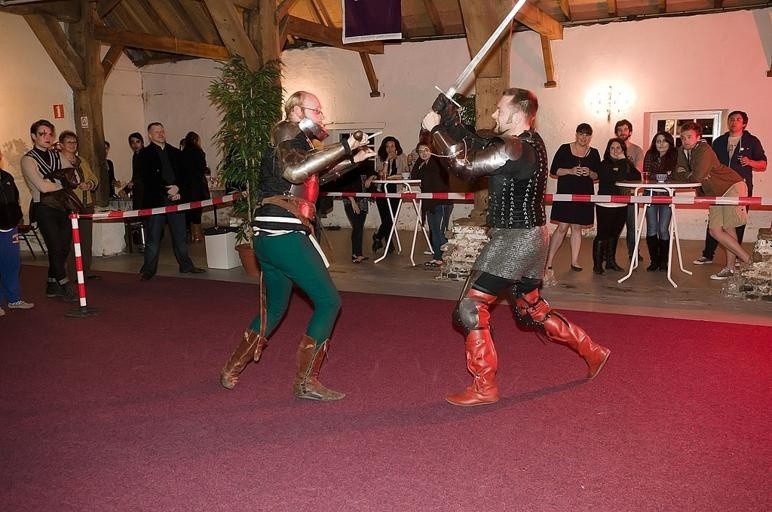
[575,144,590,167]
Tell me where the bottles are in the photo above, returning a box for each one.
[379,172,383,180]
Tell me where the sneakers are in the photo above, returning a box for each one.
[692,256,713,265]
[8,300,35,309]
[46,282,66,298]
[628,255,644,261]
[0,307,5,316]
[735,259,741,266]
[63,291,89,302]
[709,267,736,280]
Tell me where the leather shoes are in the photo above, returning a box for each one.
[140,268,154,281]
[571,264,583,272]
[180,267,206,273]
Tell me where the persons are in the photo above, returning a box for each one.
[592,138,641,274]
[20,120,121,304]
[221,92,377,402]
[341,137,451,267]
[601,119,645,262]
[643,131,679,271]
[423,87,610,407]
[1,169,34,316]
[546,123,601,271]
[693,111,767,266]
[673,122,751,281]
[118,122,211,281]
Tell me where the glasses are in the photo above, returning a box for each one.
[67,141,77,145]
[303,107,323,115]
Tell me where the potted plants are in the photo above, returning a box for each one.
[203,52,287,278]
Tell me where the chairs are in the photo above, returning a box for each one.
[17,198,46,260]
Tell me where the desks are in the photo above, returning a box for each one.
[615,180,702,288]
[371,179,435,267]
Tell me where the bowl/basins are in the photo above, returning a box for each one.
[401,172,411,180]
[656,173,667,182]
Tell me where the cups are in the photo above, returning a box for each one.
[383,172,386,180]
[642,172,650,181]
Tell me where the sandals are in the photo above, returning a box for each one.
[424,261,433,267]
[359,256,369,260]
[431,262,444,267]
[352,258,361,263]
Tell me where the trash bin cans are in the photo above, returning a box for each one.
[204,225,243,270]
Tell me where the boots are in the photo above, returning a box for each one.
[592,239,608,276]
[605,237,624,272]
[646,233,659,273]
[659,236,670,273]
[514,287,612,381]
[293,334,345,401]
[445,288,500,407]
[220,327,269,390]
